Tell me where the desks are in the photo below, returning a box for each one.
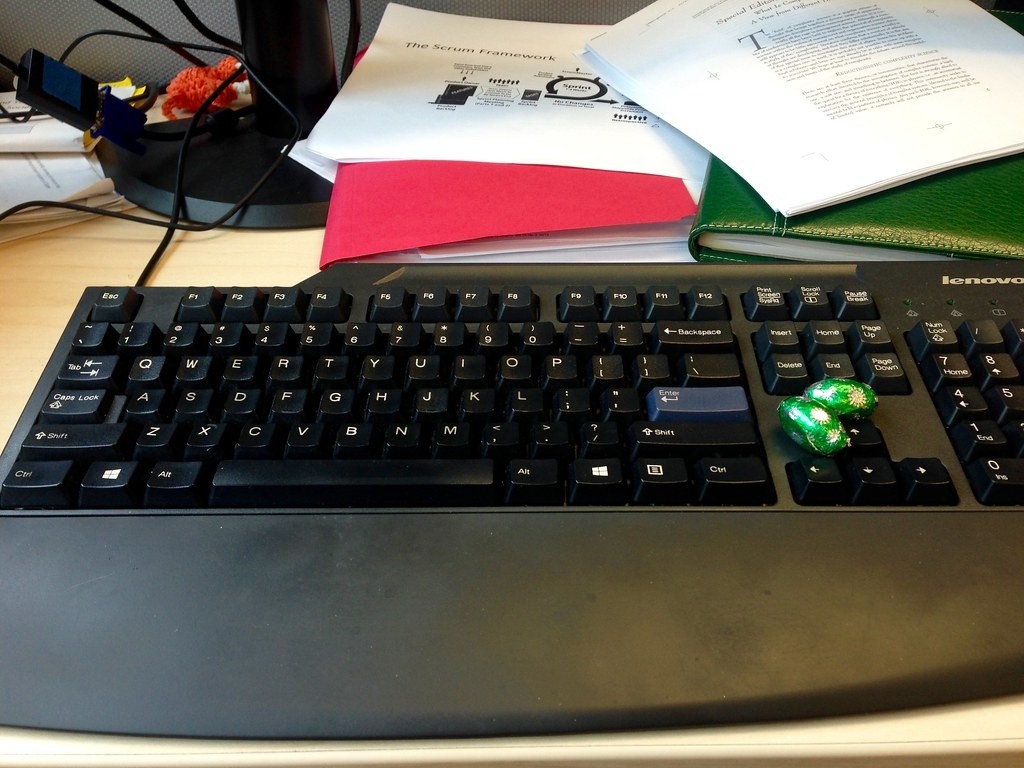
[0,93,1024,768]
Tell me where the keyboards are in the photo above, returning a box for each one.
[1,258,1023,743]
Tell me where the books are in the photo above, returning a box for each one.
[0,79,145,154]
[687,10,1024,266]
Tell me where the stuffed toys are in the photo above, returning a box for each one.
[160,58,252,121]
